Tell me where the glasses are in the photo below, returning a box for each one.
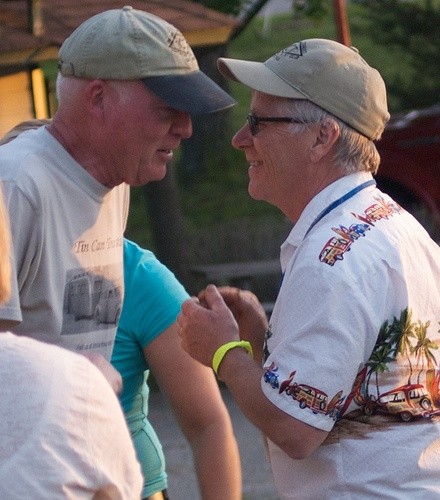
[246,114,322,135]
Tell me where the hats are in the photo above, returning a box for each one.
[217,38,390,142]
[57,6,237,114]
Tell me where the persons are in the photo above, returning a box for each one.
[0,6,243,500]
[178,39,440,500]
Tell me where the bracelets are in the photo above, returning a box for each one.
[211,340,254,382]
